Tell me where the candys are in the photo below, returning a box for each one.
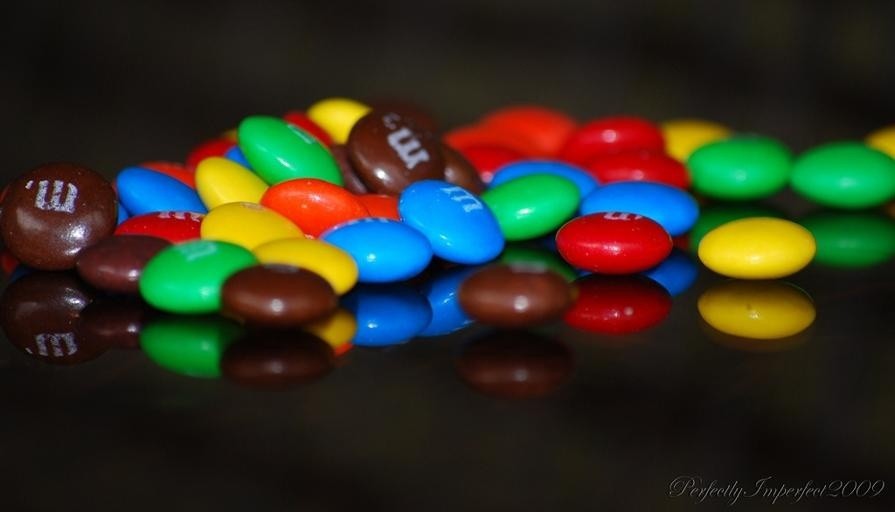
[0,97,895,402]
